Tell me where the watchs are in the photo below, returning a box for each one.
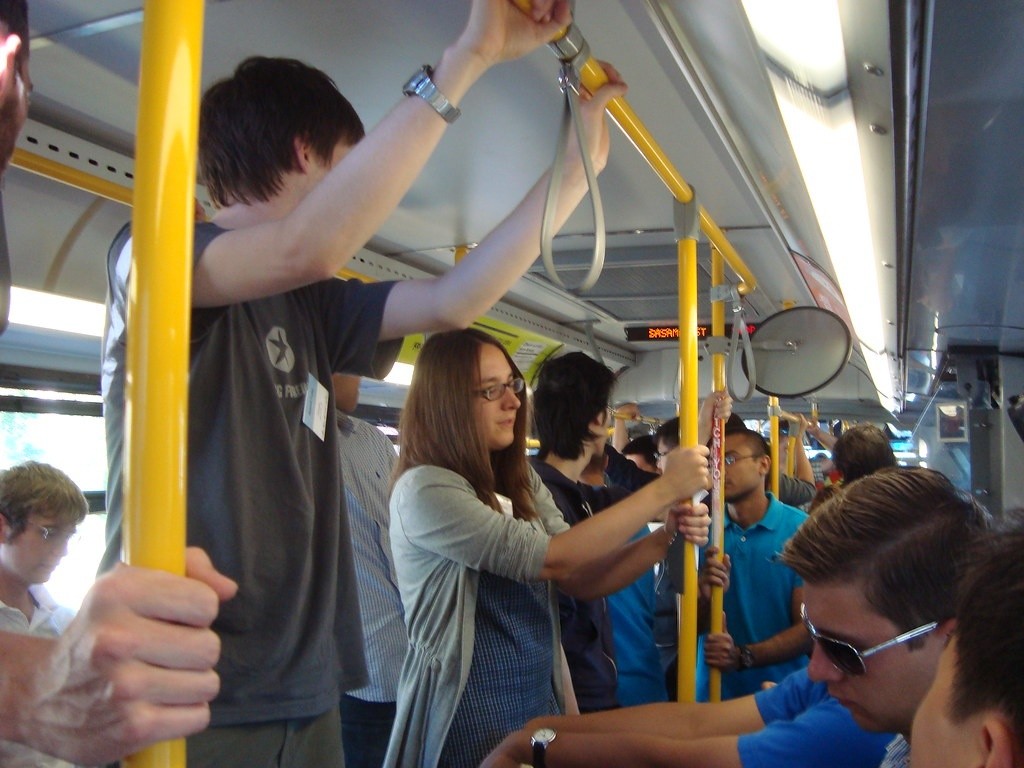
[739,646,754,671]
[403,65,461,124]
[531,727,556,768]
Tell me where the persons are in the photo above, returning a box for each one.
[1,0,238,767]
[771,419,898,515]
[911,528,1024,768]
[0,461,90,768]
[331,373,403,768]
[387,327,746,768]
[696,427,812,703]
[777,466,993,768]
[477,665,900,768]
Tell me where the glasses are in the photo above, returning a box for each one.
[653,451,669,461]
[23,521,77,543]
[725,454,761,466]
[473,377,525,401]
[799,602,938,676]
